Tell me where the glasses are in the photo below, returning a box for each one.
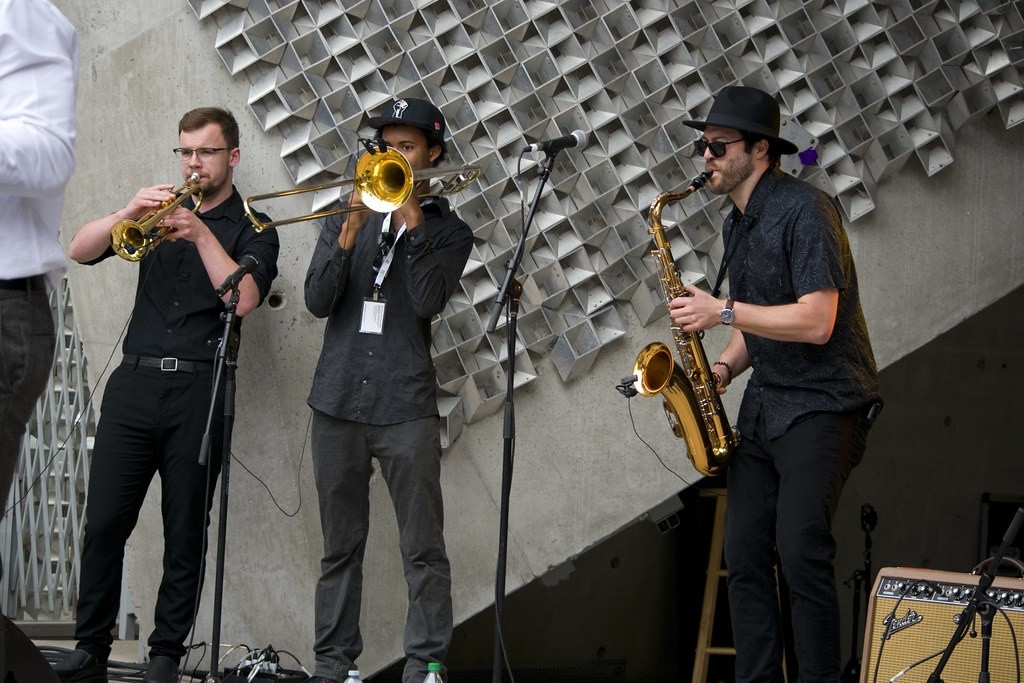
[173,148,227,160]
[694,137,744,157]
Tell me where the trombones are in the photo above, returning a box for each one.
[243,146,482,233]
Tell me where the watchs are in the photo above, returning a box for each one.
[720,298,736,327]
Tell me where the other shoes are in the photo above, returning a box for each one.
[145,656,180,683]
[52,650,107,683]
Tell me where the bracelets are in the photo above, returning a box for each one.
[714,360,734,386]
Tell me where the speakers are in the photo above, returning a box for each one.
[861,567,1023,683]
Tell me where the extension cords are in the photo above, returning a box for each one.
[244,659,278,670]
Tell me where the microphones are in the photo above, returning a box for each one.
[523,130,590,152]
[216,255,258,296]
[359,138,388,155]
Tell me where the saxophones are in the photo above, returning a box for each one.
[632,168,744,477]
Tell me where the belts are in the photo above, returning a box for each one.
[123,354,212,374]
[0,277,41,291]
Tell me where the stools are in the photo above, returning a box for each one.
[691,487,787,683]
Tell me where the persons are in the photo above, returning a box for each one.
[0,2,77,683]
[305,100,474,681]
[53,108,279,683]
[668,86,884,683]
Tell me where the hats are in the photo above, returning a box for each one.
[681,85,798,155]
[367,98,445,138]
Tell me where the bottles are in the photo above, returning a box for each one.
[344,669,362,683]
[423,662,443,683]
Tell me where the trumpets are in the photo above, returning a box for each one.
[108,171,205,262]
